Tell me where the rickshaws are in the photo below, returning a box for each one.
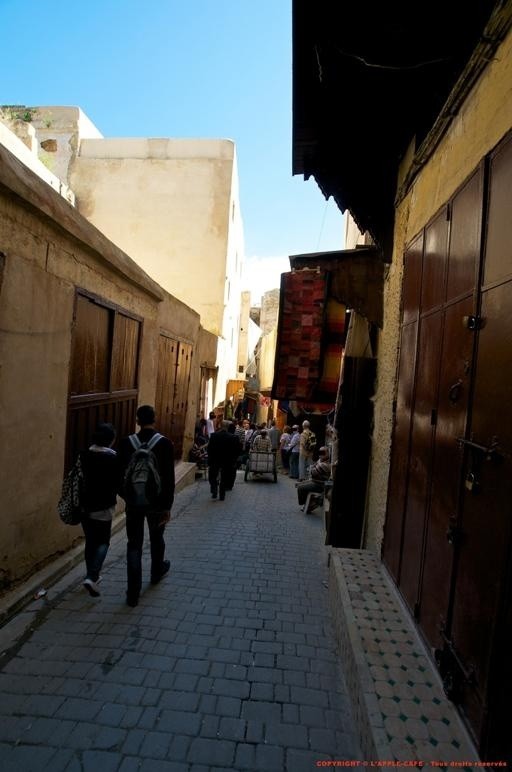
[245,451,277,481]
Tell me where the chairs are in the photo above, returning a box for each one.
[304,492,324,515]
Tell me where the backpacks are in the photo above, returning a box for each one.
[304,432,317,450]
[56,455,83,526]
[124,433,165,503]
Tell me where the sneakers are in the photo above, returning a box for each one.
[210,493,225,501]
[83,578,101,596]
[94,576,103,585]
[150,559,171,584]
[125,588,137,607]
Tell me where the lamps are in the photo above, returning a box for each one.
[345,304,351,314]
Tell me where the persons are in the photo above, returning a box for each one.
[66,419,126,600]
[115,402,176,607]
[187,396,335,515]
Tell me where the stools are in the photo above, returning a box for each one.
[198,462,207,481]
